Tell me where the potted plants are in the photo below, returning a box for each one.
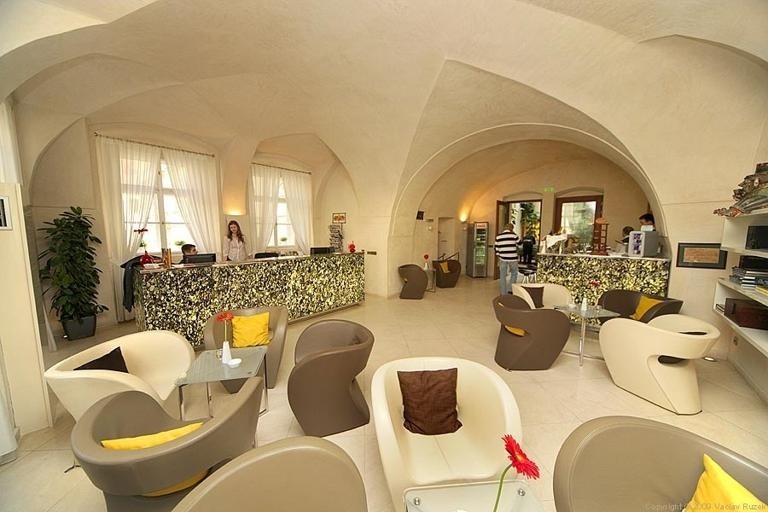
[36,206,109,341]
[175,240,185,251]
[280,237,288,245]
[137,240,147,252]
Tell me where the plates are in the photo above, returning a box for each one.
[142,263,161,270]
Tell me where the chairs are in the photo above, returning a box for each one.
[398,254,461,299]
[493,282,720,415]
[70,376,263,512]
[287,319,375,438]
[44,330,195,422]
[203,306,288,388]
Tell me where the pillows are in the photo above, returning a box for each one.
[73,346,129,374]
[102,422,209,497]
[231,312,270,347]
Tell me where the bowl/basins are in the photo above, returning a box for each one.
[227,358,241,368]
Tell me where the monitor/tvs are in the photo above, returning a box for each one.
[183,253,216,263]
[310,247,330,254]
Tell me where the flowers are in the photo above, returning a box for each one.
[217,312,234,340]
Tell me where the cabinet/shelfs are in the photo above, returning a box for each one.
[711,207,768,358]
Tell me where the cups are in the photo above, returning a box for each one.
[216,349,222,360]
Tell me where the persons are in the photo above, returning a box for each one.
[621,226,634,243]
[179,244,199,264]
[639,213,656,231]
[223,221,251,261]
[522,232,536,264]
[494,224,523,295]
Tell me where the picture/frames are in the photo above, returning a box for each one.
[676,242,728,270]
[0,196,13,230]
[332,212,346,224]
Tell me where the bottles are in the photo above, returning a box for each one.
[579,294,588,311]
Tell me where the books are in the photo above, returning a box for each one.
[729,266,768,296]
[716,304,725,315]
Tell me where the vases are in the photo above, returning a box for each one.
[222,342,231,364]
